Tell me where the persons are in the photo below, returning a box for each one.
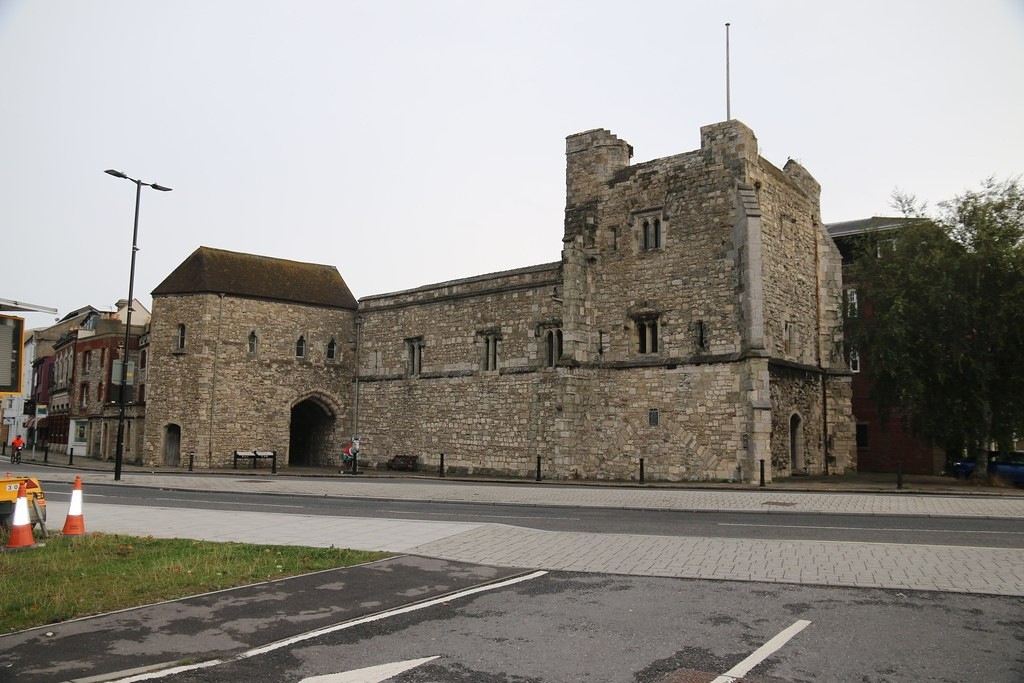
[12,434,26,459]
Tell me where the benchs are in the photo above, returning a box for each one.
[387,455,418,472]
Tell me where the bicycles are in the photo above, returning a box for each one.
[10,444,25,463]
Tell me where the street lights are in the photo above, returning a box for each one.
[104,165,172,481]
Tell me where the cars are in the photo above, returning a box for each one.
[953,447,1024,484]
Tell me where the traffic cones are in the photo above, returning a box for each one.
[59,474,88,536]
[6,481,38,549]
